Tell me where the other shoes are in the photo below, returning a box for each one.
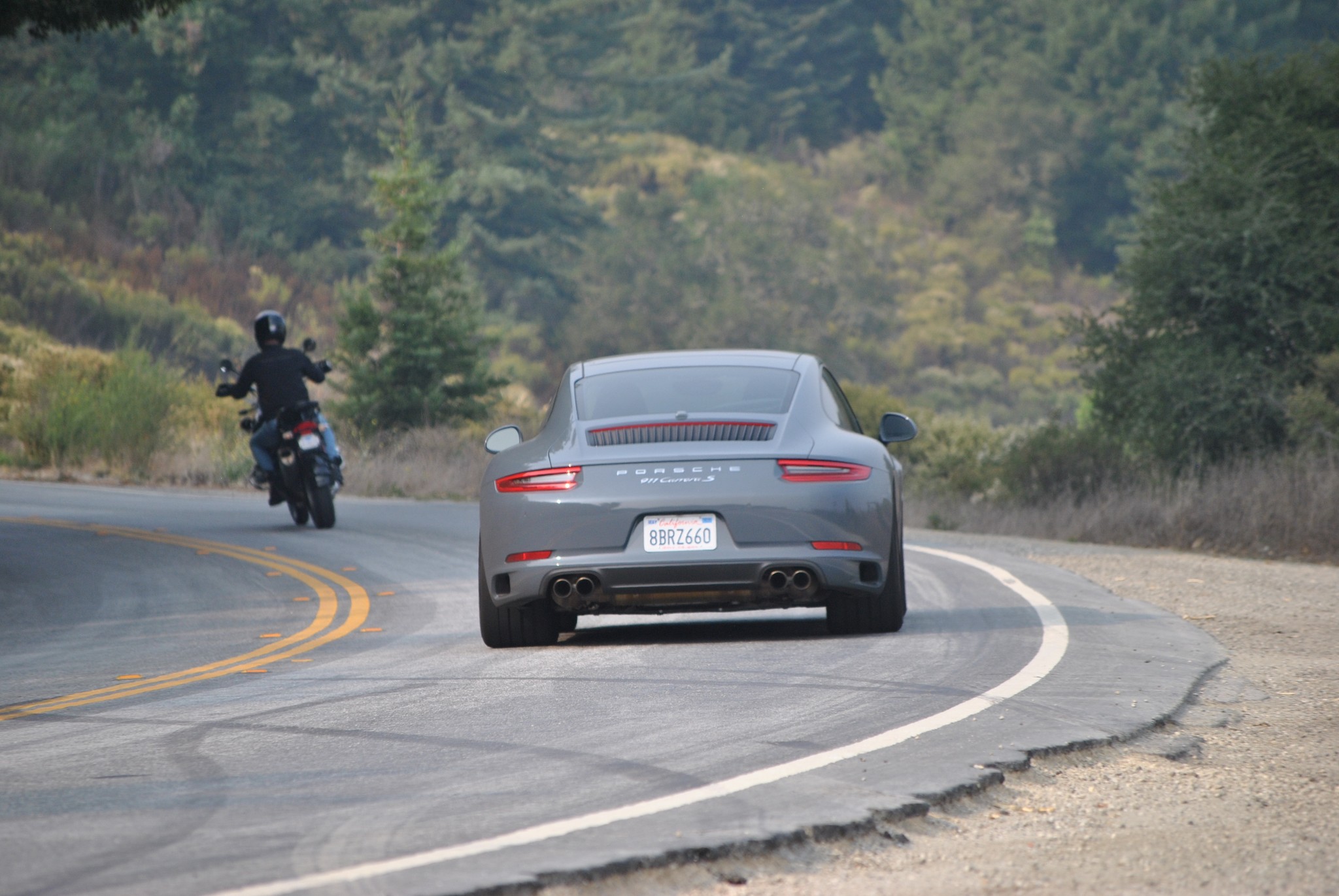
[330,454,342,468]
[269,482,286,506]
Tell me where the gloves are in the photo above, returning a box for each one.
[217,383,229,397]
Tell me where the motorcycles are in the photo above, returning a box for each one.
[218,335,345,530]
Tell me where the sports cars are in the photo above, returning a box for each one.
[477,348,918,649]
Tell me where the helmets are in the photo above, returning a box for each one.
[254,310,286,348]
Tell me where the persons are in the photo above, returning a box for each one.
[215,311,345,489]
